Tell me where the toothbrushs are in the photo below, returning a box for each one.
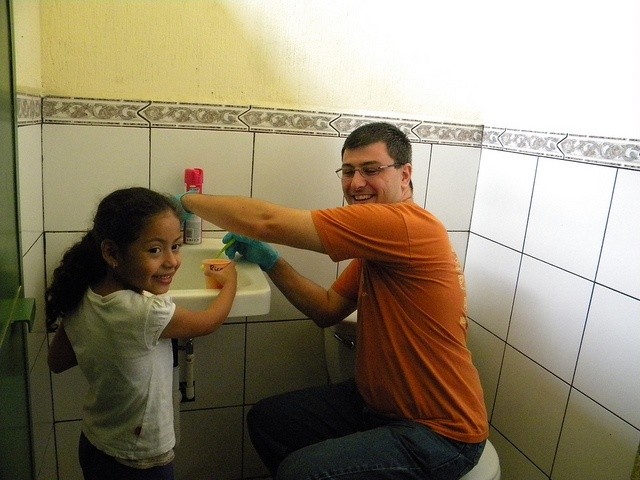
[200,240,235,270]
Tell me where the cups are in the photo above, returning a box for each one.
[201,259,232,290]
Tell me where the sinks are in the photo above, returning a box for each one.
[146,238,272,317]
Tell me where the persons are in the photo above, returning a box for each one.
[162,122,490,480]
[43,187,237,480]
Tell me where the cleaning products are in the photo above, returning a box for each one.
[183,167,203,244]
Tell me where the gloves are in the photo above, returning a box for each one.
[223,232,280,271]
[170,189,198,223]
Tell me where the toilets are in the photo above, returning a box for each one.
[324,309,501,480]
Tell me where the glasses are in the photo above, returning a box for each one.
[335,163,404,180]
